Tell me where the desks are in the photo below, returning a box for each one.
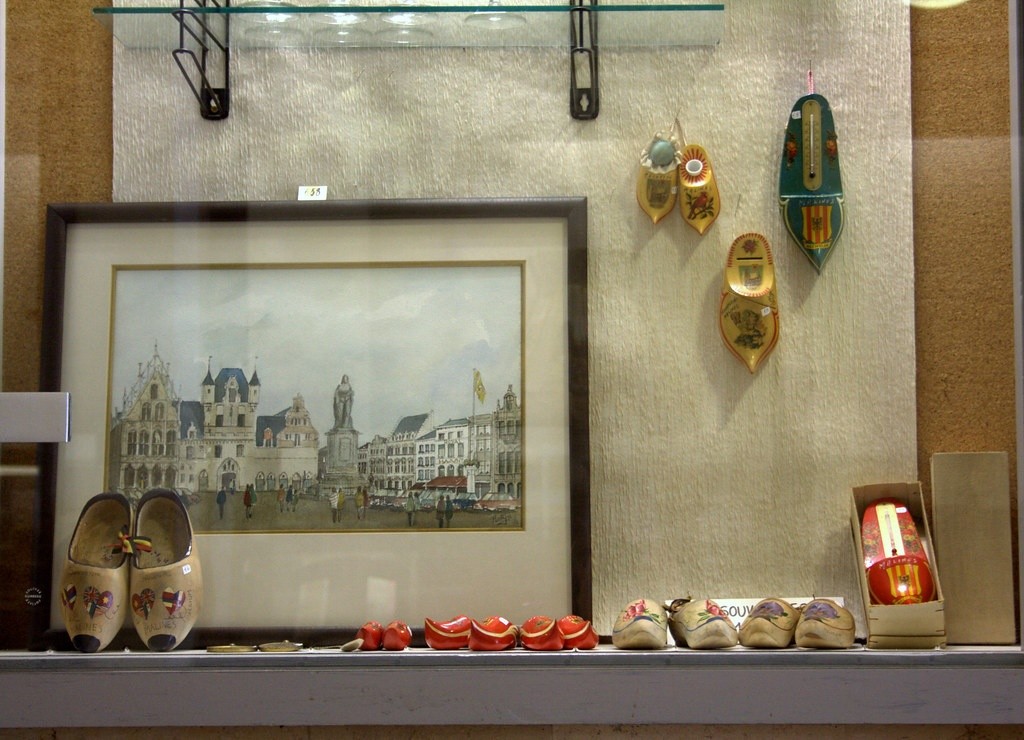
[1,644,1024,740]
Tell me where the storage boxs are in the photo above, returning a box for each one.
[849,481,948,649]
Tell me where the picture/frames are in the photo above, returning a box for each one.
[29,196,593,648]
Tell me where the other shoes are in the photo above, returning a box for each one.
[132,488,202,652]
[358,622,381,651]
[668,598,740,649]
[382,620,411,650]
[794,600,856,647]
[612,598,667,650]
[738,598,796,649]
[60,493,132,653]
